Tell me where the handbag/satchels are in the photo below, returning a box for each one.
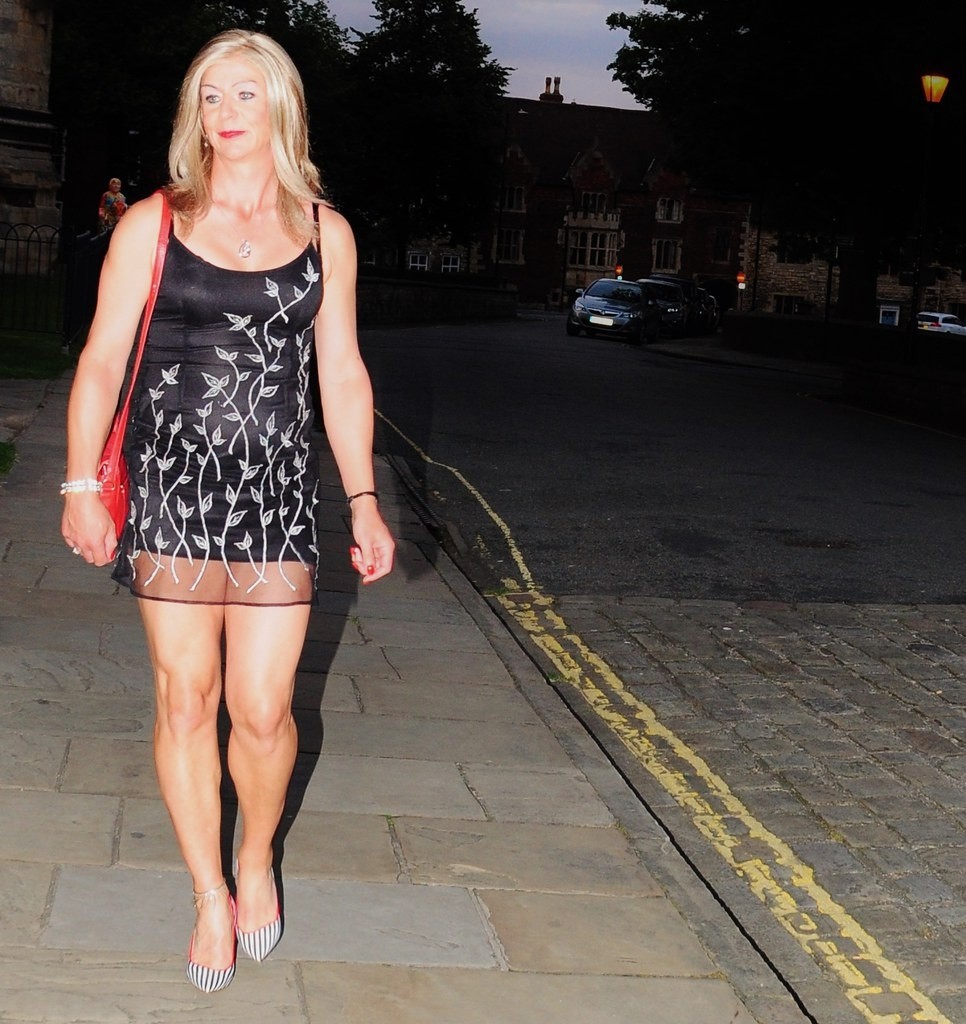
[95,409,130,561]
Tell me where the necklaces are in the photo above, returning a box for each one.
[209,196,277,259]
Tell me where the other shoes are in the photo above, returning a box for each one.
[235,867,281,962]
[185,895,236,994]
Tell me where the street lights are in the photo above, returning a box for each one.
[906,61,954,334]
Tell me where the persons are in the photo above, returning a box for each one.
[98,178,127,232]
[60,29,395,993]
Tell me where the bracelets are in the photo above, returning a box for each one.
[59,479,103,495]
[347,492,380,506]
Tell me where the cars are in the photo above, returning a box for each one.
[637,277,691,339]
[915,311,966,337]
[568,277,663,347]
[652,273,722,337]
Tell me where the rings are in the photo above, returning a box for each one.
[71,548,81,556]
